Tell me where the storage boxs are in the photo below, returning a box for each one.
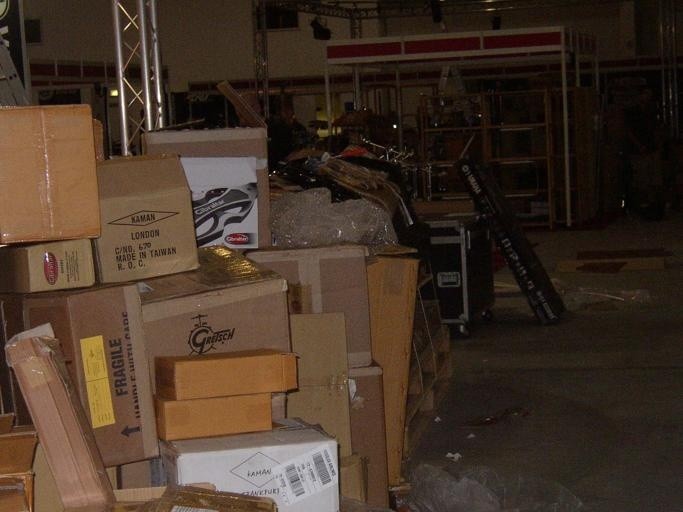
[1,104,423,512]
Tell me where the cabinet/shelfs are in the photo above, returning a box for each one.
[418,87,557,231]
